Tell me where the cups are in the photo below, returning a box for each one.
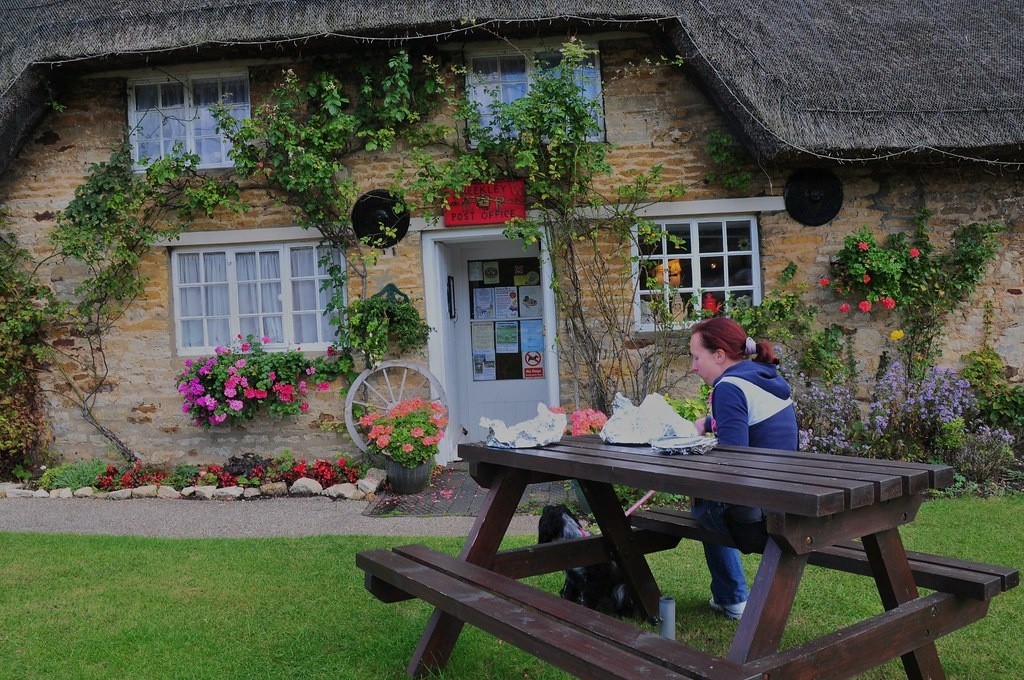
[658,596,675,641]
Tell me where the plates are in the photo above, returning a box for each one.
[649,437,716,450]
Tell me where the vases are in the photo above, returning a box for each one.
[571,480,596,514]
[385,454,431,494]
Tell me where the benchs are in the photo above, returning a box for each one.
[356,544,767,680]
[631,505,1018,601]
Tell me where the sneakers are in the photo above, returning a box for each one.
[709,596,746,619]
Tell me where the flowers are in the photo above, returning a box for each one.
[552,406,608,437]
[174,335,335,425]
[358,398,447,468]
[817,242,920,314]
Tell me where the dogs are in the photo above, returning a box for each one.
[538,504,641,619]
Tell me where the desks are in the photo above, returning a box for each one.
[407,443,953,680]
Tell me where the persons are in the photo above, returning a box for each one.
[690,315,800,619]
